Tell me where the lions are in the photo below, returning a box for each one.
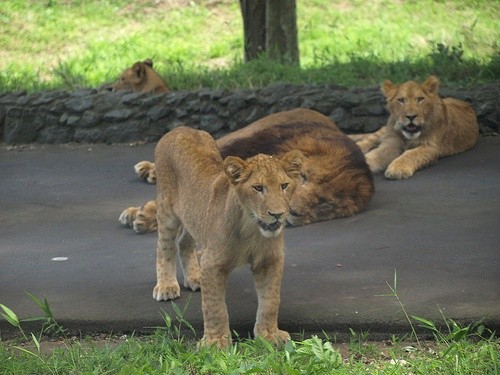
[118,107,377,234]
[152,126,304,353]
[102,58,174,94]
[345,77,479,180]
